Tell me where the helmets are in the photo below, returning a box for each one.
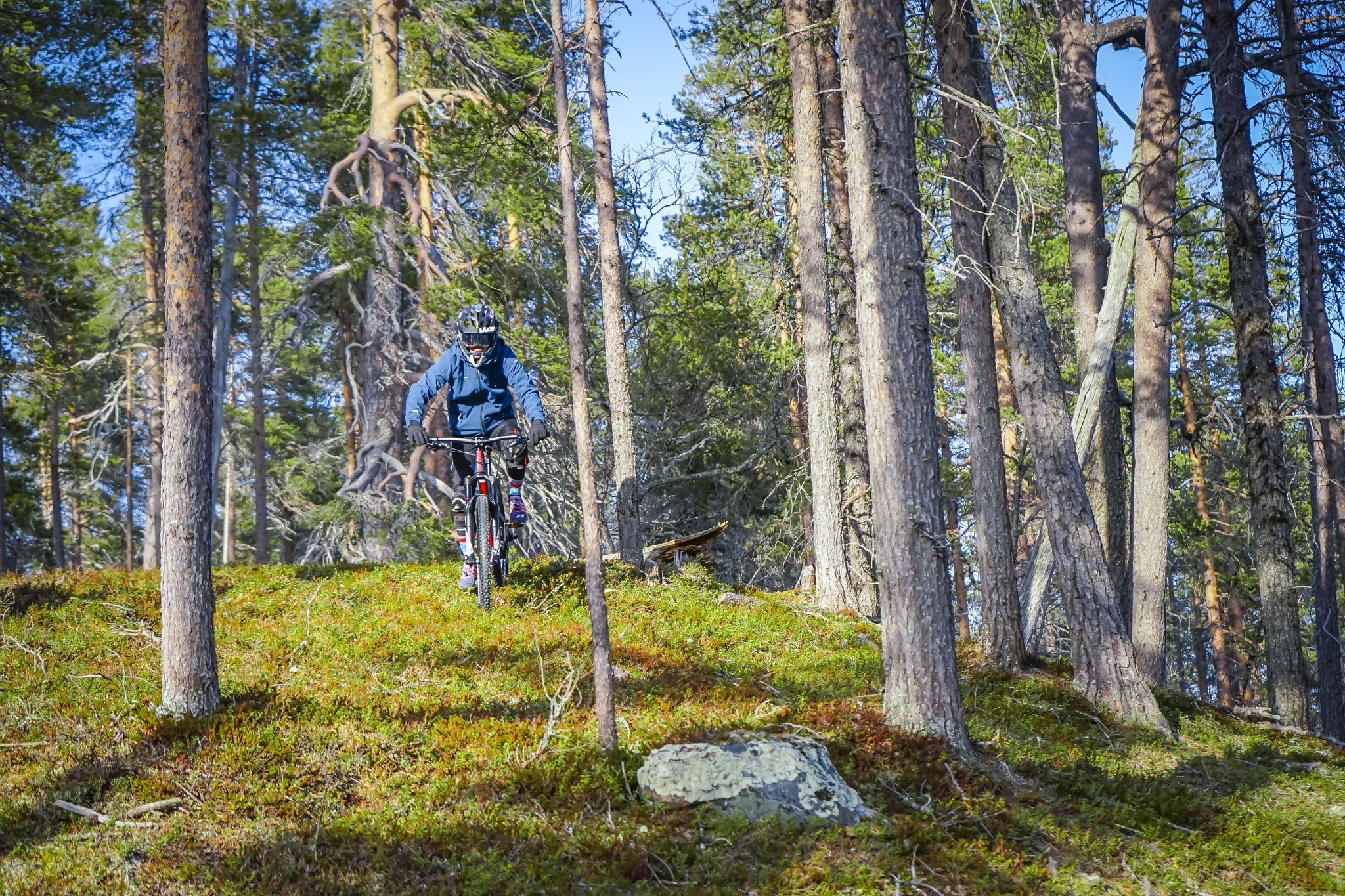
[455,303,500,369]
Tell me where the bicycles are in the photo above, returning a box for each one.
[406,428,552,614]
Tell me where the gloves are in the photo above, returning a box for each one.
[407,420,430,447]
[529,419,548,447]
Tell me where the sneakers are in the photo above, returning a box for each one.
[508,493,528,526]
[458,561,479,591]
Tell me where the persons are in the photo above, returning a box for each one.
[405,304,546,593]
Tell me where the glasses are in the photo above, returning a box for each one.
[463,332,496,346]
